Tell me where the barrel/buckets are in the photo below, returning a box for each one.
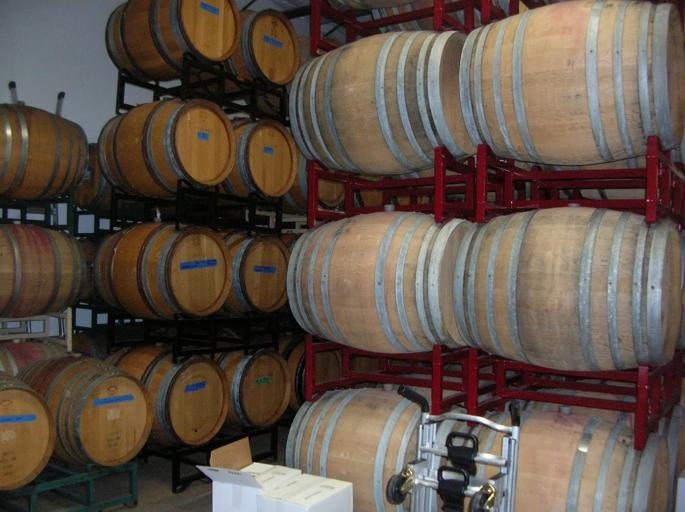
[456,0,681,171]
[283,387,423,511]
[287,210,467,357]
[96,220,300,327]
[430,407,685,512]
[289,28,472,177]
[2,338,338,492]
[453,203,683,373]
[99,2,298,100]
[95,97,300,206]
[1,103,89,201]
[1,224,86,314]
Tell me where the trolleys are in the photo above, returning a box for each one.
[383,386,521,512]
